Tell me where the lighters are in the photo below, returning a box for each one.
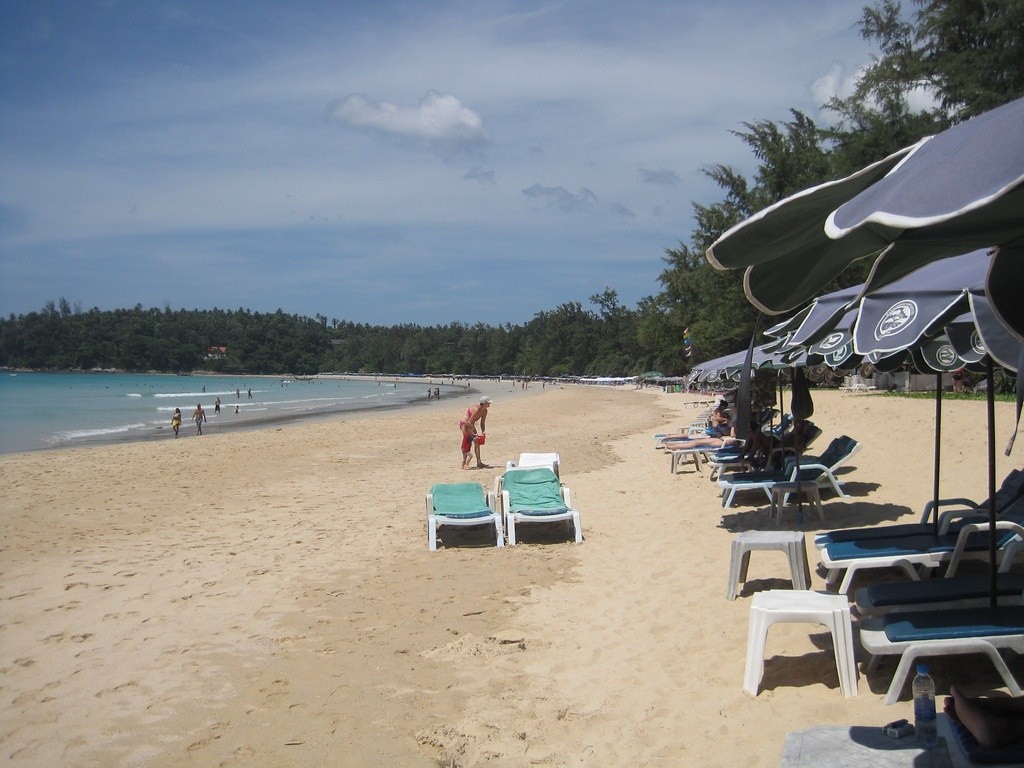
[887,719,909,728]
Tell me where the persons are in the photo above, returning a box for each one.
[459,396,493,467]
[171,385,254,436]
[952,366,964,395]
[948,686,1024,749]
[461,422,479,470]
[665,399,803,465]
[427,377,545,399]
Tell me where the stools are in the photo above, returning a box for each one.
[771,481,826,526]
[728,530,812,602]
[697,450,711,464]
[743,588,857,700]
[671,450,701,473]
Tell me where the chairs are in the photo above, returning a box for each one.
[653,375,1024,768]
[426,482,505,551]
[495,453,583,544]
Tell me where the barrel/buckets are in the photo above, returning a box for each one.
[477,434,486,445]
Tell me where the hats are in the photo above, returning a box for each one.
[479,396,493,405]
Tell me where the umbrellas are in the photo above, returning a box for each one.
[580,371,664,381]
[689,97,1024,625]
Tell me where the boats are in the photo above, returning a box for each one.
[294,376,313,380]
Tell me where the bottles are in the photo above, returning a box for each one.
[912,665,938,747]
